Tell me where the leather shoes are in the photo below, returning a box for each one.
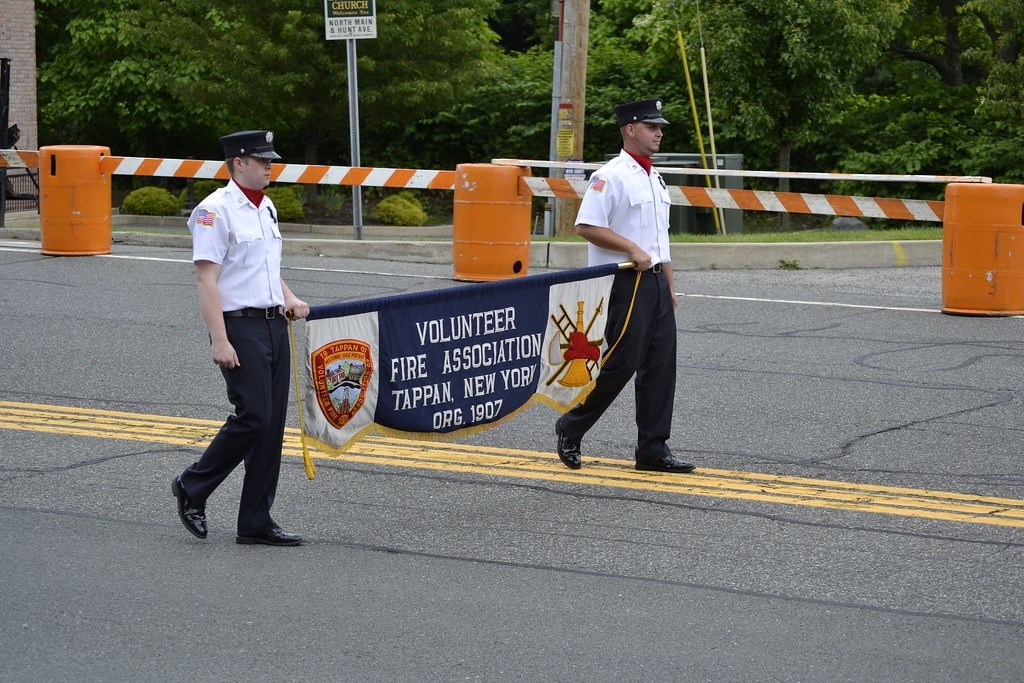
[171,476,208,538]
[555,418,582,469]
[235,527,303,545]
[635,454,696,472]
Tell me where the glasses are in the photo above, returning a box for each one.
[245,155,272,164]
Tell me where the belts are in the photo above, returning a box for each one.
[223,306,278,319]
[651,263,663,273]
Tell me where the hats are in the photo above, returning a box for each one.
[614,98,670,124]
[219,130,281,159]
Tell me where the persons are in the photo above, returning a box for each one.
[171,130,310,546]
[556,98,695,473]
